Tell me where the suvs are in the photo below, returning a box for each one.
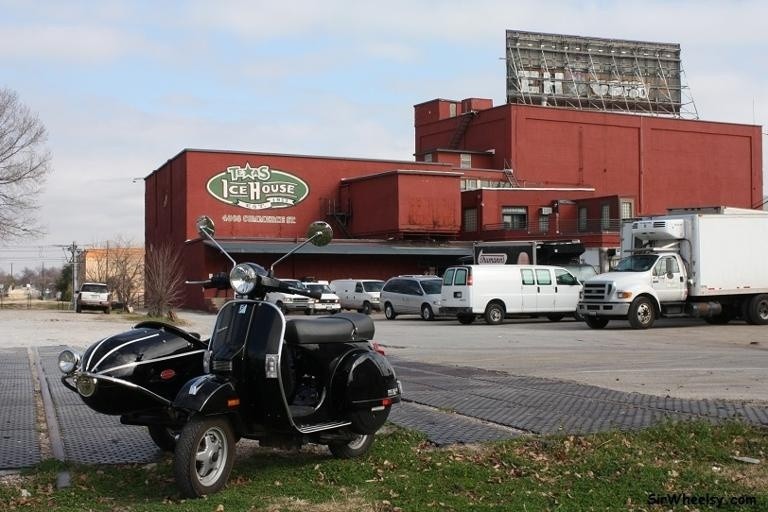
[379,272,457,321]
[264,276,314,315]
[76,282,113,316]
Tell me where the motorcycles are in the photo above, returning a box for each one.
[57,215,404,501]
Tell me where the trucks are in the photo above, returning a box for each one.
[575,203,767,330]
[329,279,389,314]
[440,263,587,325]
[472,237,597,284]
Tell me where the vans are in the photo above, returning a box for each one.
[299,281,341,316]
